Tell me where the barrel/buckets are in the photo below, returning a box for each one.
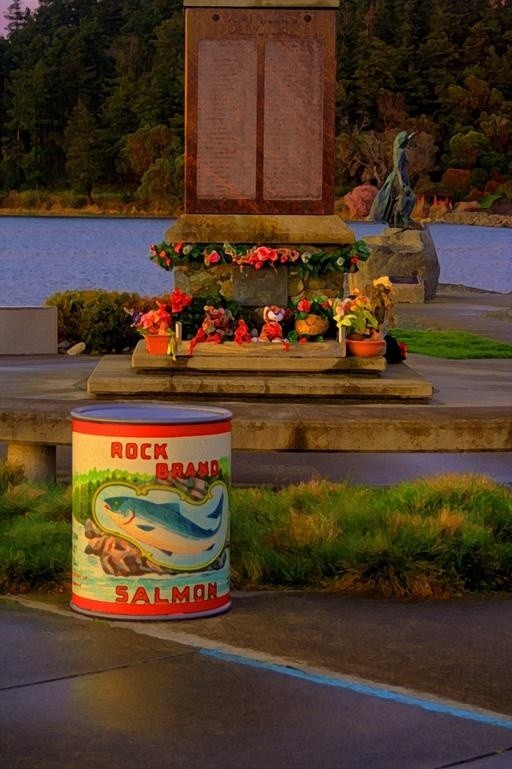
[68,401,233,621]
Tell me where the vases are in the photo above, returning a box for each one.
[142,333,170,355]
[345,339,385,358]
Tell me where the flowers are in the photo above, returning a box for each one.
[123,289,192,336]
[327,291,380,336]
[147,238,358,275]
